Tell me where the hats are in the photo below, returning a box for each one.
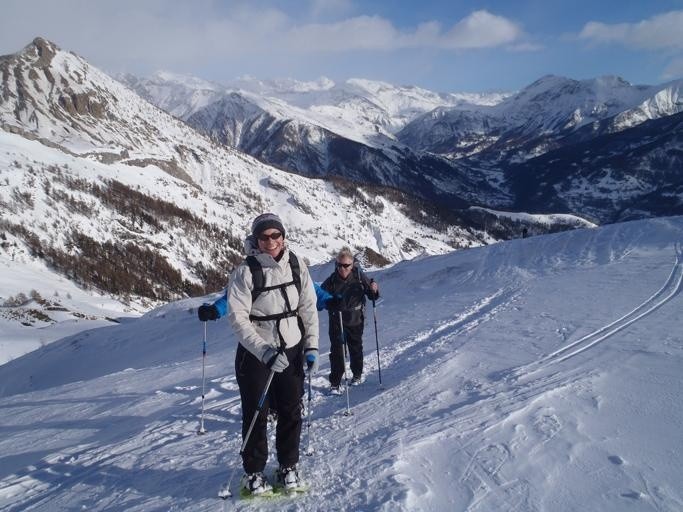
[251,213,285,240]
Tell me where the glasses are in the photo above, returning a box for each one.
[258,232,282,241]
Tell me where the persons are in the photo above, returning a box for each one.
[228,211,321,497]
[315,245,381,399]
[196,279,349,430]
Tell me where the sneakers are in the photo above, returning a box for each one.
[242,473,272,494]
[353,373,361,385]
[278,464,303,488]
[330,383,341,394]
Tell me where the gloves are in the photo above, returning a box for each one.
[198,303,220,321]
[262,348,289,373]
[328,295,346,312]
[302,349,319,377]
